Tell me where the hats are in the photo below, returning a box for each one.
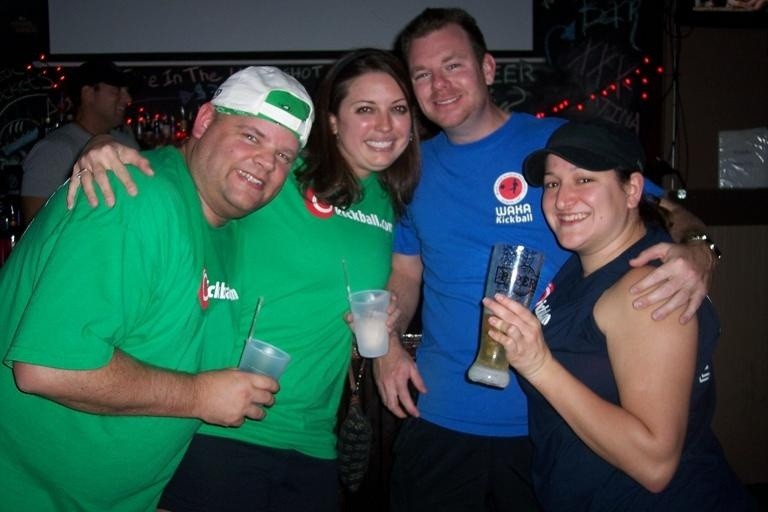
[68,59,136,89]
[522,115,646,188]
[209,65,316,151]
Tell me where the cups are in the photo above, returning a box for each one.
[233,336,290,382]
[465,239,543,391]
[343,290,391,358]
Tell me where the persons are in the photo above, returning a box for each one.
[1,67,315,512]
[371,5,724,512]
[69,49,421,511]
[482,115,755,512]
[21,59,138,231]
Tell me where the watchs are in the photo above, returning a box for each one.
[685,226,726,265]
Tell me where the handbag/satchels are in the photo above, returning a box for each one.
[337,351,371,493]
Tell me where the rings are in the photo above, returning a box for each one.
[77,168,93,178]
